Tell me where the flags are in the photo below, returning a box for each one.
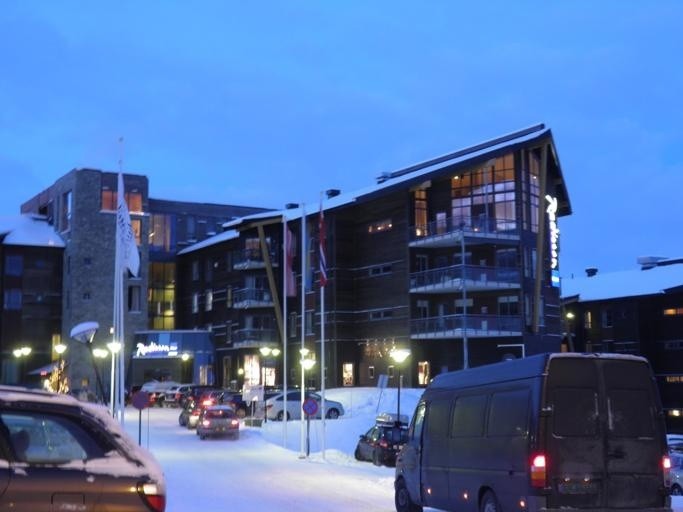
[301,207,312,290]
[117,171,140,277]
[282,216,298,297]
[318,201,329,286]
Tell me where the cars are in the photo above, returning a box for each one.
[141,381,277,440]
[262,390,345,419]
[667,434,683,494]
[355,423,410,468]
[1,384,167,512]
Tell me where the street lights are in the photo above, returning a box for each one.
[13,345,32,385]
[391,348,410,421]
[299,347,316,455]
[54,344,66,393]
[261,347,280,421]
[92,342,122,418]
[70,321,113,416]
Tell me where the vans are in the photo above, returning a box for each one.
[393,353,673,510]
